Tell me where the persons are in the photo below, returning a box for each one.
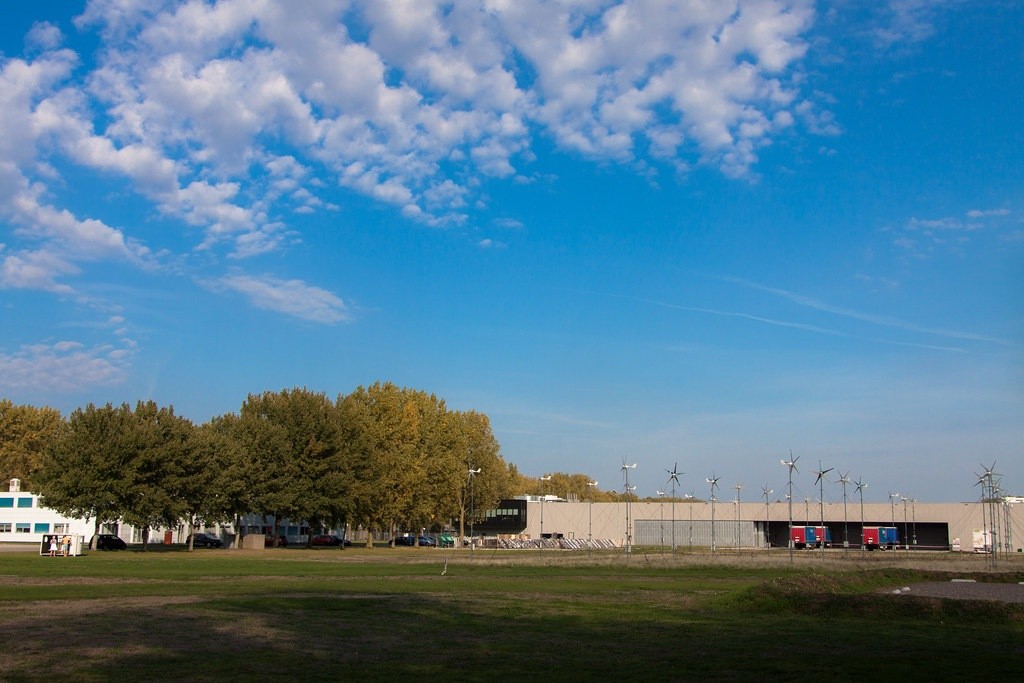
[61,535,71,556]
[50,536,58,557]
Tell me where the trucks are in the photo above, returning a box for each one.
[972,528,994,553]
[862,527,900,551]
[790,526,831,550]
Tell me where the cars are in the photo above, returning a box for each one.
[332,535,351,546]
[313,535,336,547]
[413,535,468,547]
[265,535,288,548]
[89,534,127,551]
[388,537,412,546]
[1002,499,1021,504]
[186,533,223,549]
